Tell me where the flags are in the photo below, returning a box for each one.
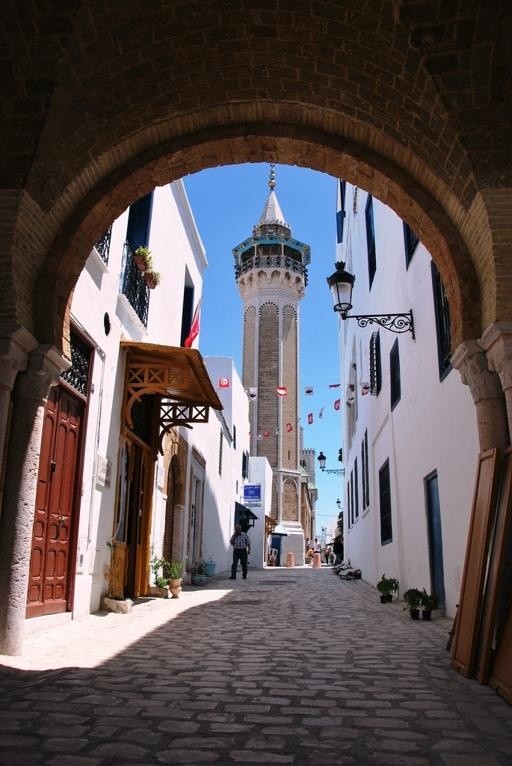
[184,297,199,348]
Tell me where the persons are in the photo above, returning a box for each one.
[324,546,330,564]
[314,539,321,553]
[228,526,250,580]
[305,538,309,551]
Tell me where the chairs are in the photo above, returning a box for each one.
[267,547,278,565]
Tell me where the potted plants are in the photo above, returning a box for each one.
[404,588,421,620]
[377,573,391,602]
[133,245,152,273]
[420,588,441,619]
[143,267,161,289]
[165,558,185,598]
[191,562,207,586]
[386,577,398,601]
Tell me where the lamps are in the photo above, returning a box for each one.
[317,451,345,476]
[323,260,416,346]
[336,497,342,509]
